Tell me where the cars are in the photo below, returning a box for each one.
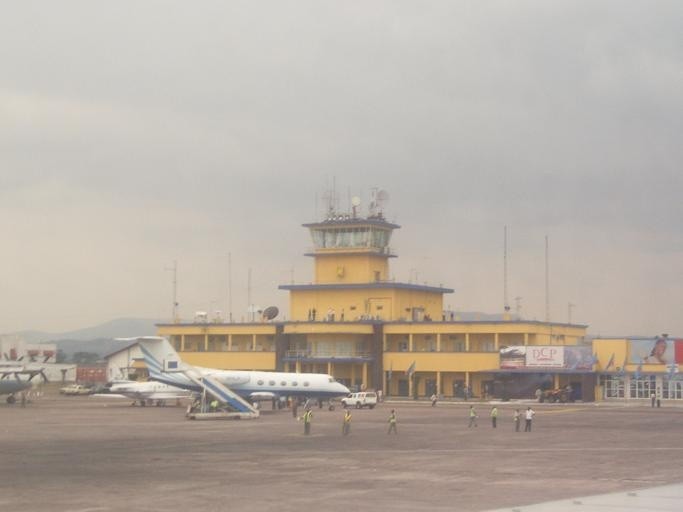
[60,385,91,395]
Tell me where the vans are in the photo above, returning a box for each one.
[341,392,378,409]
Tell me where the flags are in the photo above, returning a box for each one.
[604,354,615,373]
[403,362,417,376]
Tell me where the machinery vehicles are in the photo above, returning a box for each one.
[545,387,571,403]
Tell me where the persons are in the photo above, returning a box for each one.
[642,339,666,364]
[514,385,576,431]
[386,409,398,435]
[651,391,660,408]
[431,392,436,406]
[489,406,497,428]
[192,395,352,437]
[468,405,479,429]
[327,306,379,322]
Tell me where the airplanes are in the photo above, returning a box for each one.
[114,335,350,411]
[0,367,47,404]
[109,379,191,407]
